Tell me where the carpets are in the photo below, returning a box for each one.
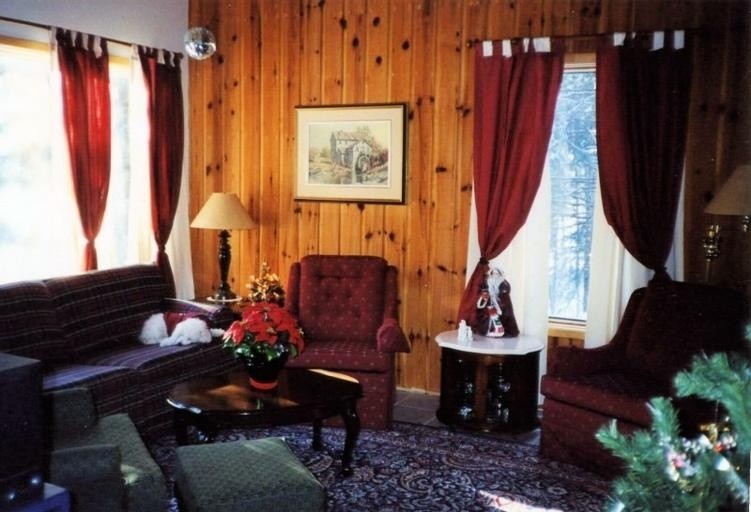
[138,417,619,512]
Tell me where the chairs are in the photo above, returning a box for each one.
[540,286,751,479]
[283,254,410,430]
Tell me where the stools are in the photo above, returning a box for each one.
[173,437,327,512]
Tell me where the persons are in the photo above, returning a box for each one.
[468,266,520,338]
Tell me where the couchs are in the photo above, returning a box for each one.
[1,262,243,435]
[45,388,169,511]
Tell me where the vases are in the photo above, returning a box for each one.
[249,358,282,389]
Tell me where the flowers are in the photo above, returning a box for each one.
[222,301,306,366]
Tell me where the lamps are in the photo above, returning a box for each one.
[704,165,751,236]
[189,192,257,300]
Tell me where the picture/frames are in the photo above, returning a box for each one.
[294,101,408,205]
